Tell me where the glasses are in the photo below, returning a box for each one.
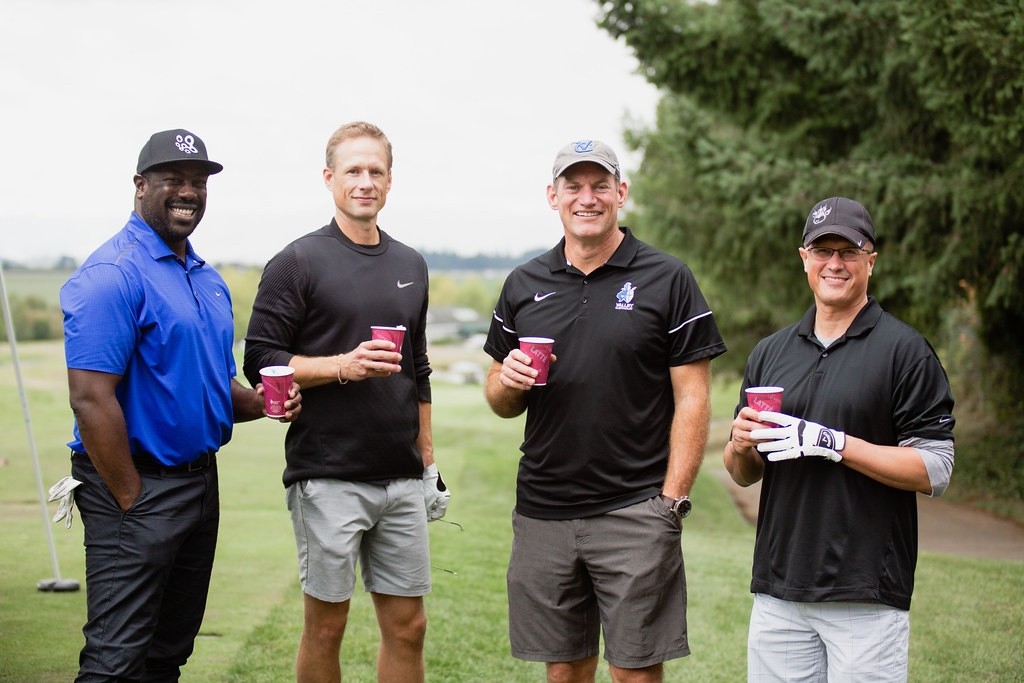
[807,246,870,262]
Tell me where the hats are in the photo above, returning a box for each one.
[802,196,876,249]
[553,140,620,181]
[137,129,223,175]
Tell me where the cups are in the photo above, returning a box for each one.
[371,325,407,365]
[259,365,296,418]
[518,337,555,386]
[745,386,784,428]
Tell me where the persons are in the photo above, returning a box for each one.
[722,196,956,683]
[482,138,727,683]
[59,128,304,682]
[243,119,452,683]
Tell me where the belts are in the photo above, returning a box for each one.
[135,453,215,473]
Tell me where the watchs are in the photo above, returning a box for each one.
[661,493,695,518]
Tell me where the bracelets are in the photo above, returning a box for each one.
[337,353,349,384]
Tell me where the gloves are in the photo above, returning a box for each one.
[750,410,846,462]
[423,462,450,522]
[48,477,83,529]
